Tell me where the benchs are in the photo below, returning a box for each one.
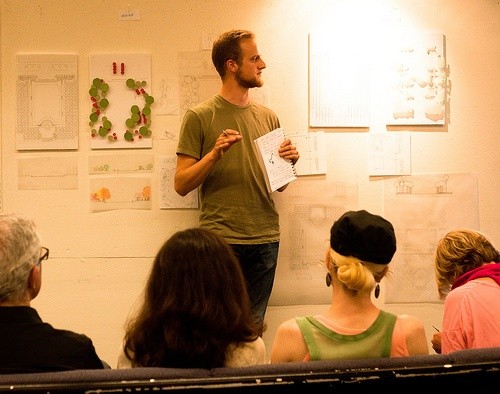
[0,348,500,394]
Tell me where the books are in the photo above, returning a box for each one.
[253,127,298,193]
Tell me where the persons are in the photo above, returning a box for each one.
[1,214,104,374]
[116,229,266,369]
[431,230,500,355]
[173,30,300,333]
[270,209,429,364]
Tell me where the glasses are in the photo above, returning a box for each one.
[28,247,49,287]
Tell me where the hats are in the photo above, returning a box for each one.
[330,210,396,264]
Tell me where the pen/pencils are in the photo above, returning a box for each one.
[222,130,228,136]
